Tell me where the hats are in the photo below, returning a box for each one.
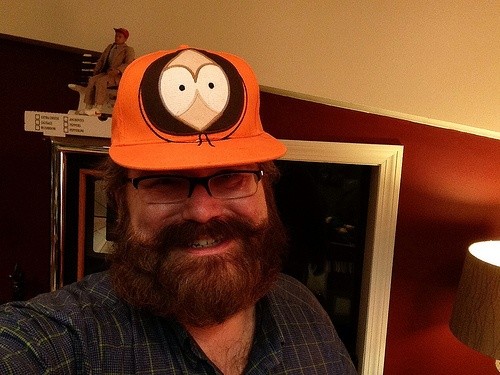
[107,42,288,168]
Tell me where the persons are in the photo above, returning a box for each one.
[3,46,362,375]
[75,28,136,116]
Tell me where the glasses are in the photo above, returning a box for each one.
[123,168,268,204]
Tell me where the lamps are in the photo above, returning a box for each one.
[448,240,500,360]
